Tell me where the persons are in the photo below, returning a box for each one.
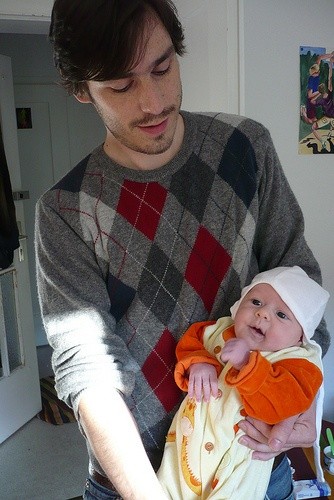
[32,0,333,500]
[149,265,331,500]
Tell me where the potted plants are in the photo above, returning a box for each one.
[323,427,334,476]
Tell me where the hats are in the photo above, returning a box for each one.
[230,264,330,347]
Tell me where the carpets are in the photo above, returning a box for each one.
[35,374,79,426]
[285,419,334,500]
